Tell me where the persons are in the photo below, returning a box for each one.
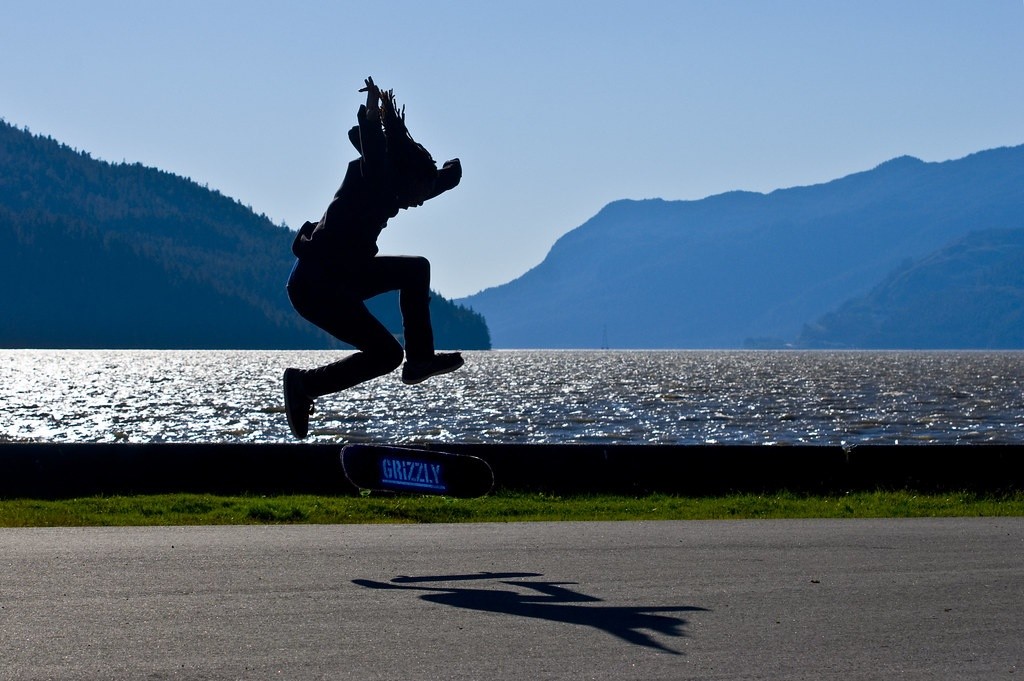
[282,76,465,440]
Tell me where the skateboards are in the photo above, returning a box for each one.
[339,443,494,499]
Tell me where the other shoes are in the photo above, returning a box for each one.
[283,366,319,440]
[402,351,465,386]
